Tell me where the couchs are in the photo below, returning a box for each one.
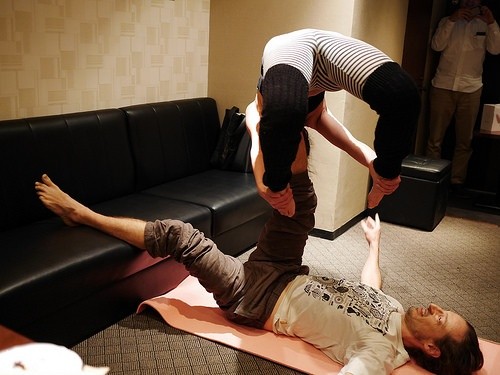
[0,96,274,350]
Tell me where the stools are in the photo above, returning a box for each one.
[366,155,451,232]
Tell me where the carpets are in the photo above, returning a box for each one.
[134,270,499,374]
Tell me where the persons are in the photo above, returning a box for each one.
[34,128,483,374]
[425,0,500,192]
[240,27,422,217]
